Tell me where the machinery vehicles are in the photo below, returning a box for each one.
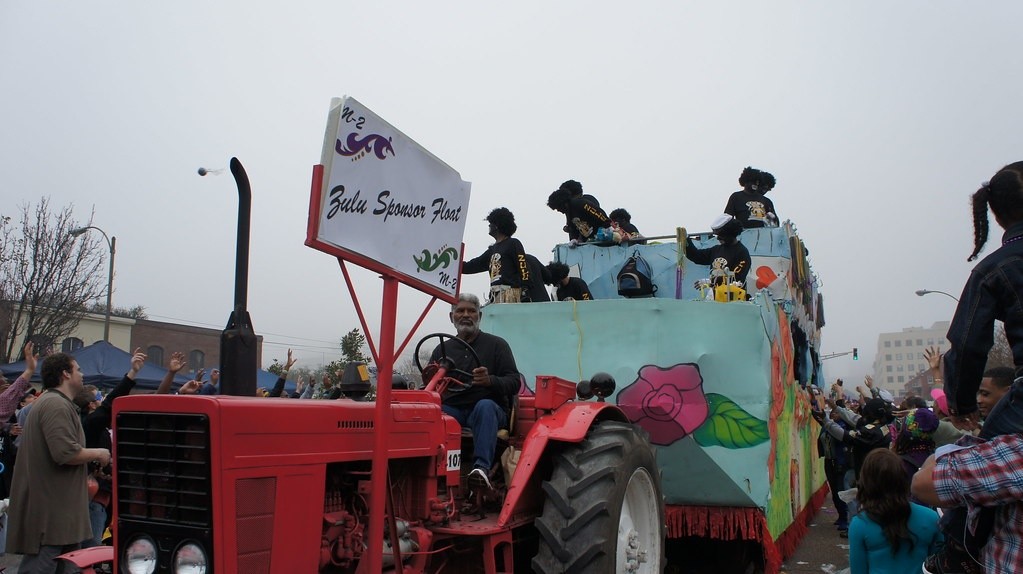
[51,153,671,573]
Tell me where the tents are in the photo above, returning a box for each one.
[186,365,305,396]
[0,341,192,390]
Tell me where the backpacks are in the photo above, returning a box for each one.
[616,248,657,296]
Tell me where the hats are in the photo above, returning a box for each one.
[931,388,949,417]
[17,387,36,409]
[876,389,894,404]
[711,211,733,233]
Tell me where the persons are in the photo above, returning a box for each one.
[548,180,640,247]
[724,168,780,227]
[545,262,594,300]
[677,215,750,298]
[257,348,343,399]
[428,294,521,502]
[0,340,220,574]
[807,159,1023,574]
[409,382,415,389]
[523,254,551,301]
[462,208,526,302]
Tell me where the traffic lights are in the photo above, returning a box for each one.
[853,347,858,359]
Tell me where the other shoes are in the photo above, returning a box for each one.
[922,545,980,574]
[835,516,847,529]
[468,469,491,508]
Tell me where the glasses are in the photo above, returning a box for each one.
[87,391,103,406]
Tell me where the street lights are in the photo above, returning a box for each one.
[68,226,116,344]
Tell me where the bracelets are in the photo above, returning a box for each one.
[282,370,288,374]
[935,379,941,381]
